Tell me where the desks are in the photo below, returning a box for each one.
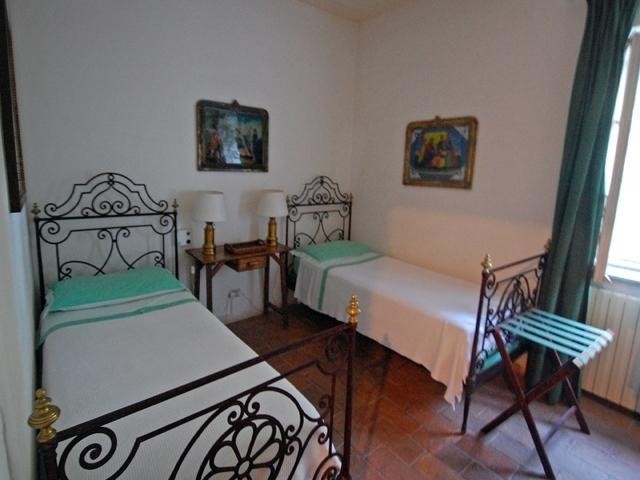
[185,237,292,331]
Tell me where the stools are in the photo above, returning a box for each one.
[481,307,615,480]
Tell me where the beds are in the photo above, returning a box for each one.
[285,174,555,409]
[28,172,362,479]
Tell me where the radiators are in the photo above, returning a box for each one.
[580,284,640,420]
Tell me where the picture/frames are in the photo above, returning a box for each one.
[0,0,27,213]
[195,100,269,172]
[402,115,479,189]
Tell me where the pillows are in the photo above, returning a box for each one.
[46,265,186,315]
[297,239,370,262]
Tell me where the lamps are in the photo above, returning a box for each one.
[255,187,288,248]
[194,189,227,255]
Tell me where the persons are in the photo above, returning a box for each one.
[206,124,262,163]
[414,133,461,172]
